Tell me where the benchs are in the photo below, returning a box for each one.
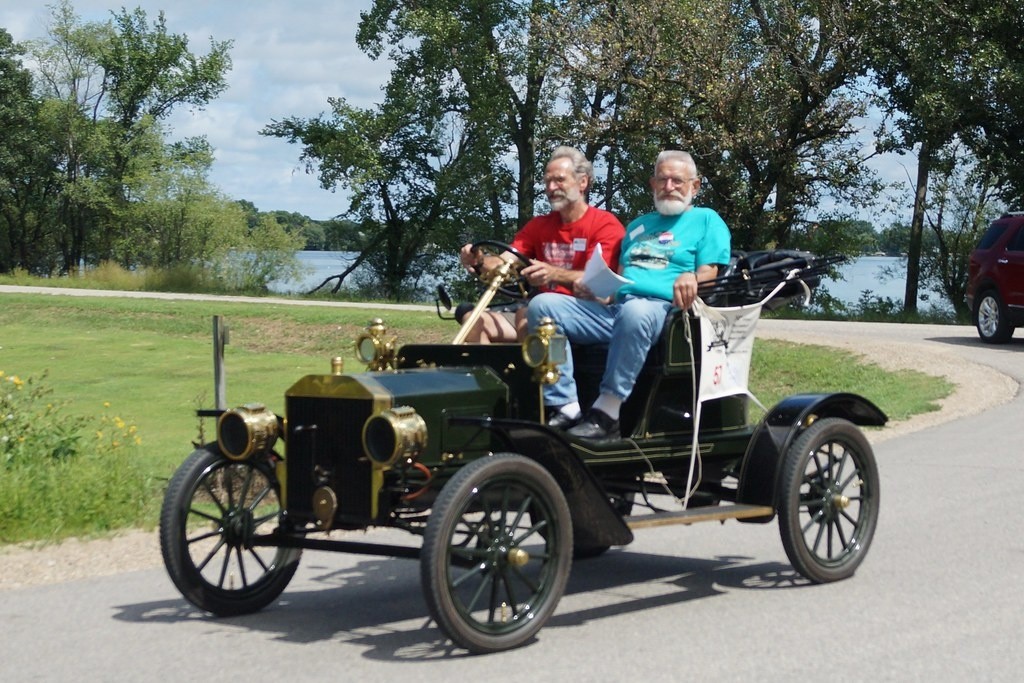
[570,249,824,383]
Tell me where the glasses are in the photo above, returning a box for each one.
[656,177,693,187]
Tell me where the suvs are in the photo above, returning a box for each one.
[964,212,1024,344]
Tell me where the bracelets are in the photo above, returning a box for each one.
[474,257,483,271]
[689,271,697,281]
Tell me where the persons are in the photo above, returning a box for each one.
[527,151,731,444]
[461,146,626,344]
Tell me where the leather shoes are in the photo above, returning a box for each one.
[545,408,620,439]
